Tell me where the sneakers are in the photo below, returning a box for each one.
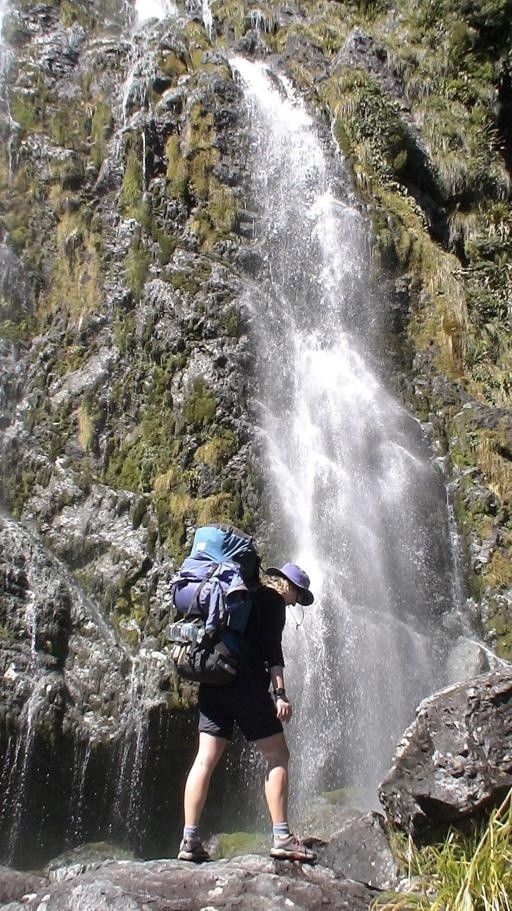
[269,834,318,861]
[178,837,209,862]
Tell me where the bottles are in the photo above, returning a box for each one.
[164,622,206,643]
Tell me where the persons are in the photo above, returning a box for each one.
[178,562,317,862]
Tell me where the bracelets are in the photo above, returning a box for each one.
[275,695,285,701]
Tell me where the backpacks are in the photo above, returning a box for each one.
[167,524,259,683]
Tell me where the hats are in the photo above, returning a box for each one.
[266,564,313,606]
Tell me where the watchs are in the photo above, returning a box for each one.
[273,688,285,695]
